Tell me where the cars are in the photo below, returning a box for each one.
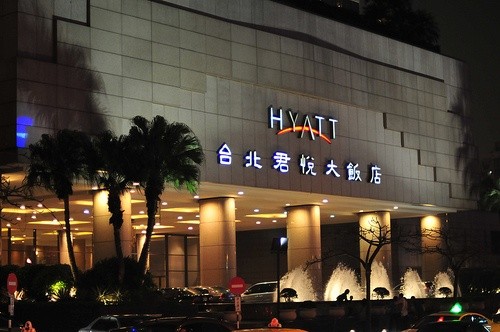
[396,293,500,332]
[80,311,307,332]
[149,280,234,304]
[241,280,280,303]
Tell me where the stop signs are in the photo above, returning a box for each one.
[7,273,17,294]
[229,276,245,295]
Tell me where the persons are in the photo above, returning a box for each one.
[336,289,350,304]
[389,296,402,331]
[398,293,409,330]
[408,295,416,322]
